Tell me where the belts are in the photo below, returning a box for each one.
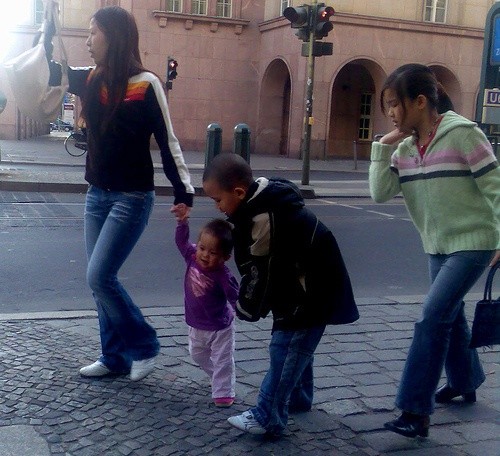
[383,409,429,438]
[432,381,477,404]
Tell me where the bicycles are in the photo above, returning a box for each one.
[64,128,87,157]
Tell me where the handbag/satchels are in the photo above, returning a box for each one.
[468,260,500,350]
[4,1,69,123]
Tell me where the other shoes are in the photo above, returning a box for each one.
[213,396,234,406]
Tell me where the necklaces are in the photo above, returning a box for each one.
[420,114,439,149]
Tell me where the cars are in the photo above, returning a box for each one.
[49,118,73,132]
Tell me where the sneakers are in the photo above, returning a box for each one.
[227,410,267,435]
[130,356,155,382]
[80,359,111,376]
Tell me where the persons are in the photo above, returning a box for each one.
[169,202,240,407]
[202,152,361,437]
[32,0,196,382]
[368,63,500,438]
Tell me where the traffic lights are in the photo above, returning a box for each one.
[315,3,334,38]
[283,5,309,27]
[167,58,178,81]
